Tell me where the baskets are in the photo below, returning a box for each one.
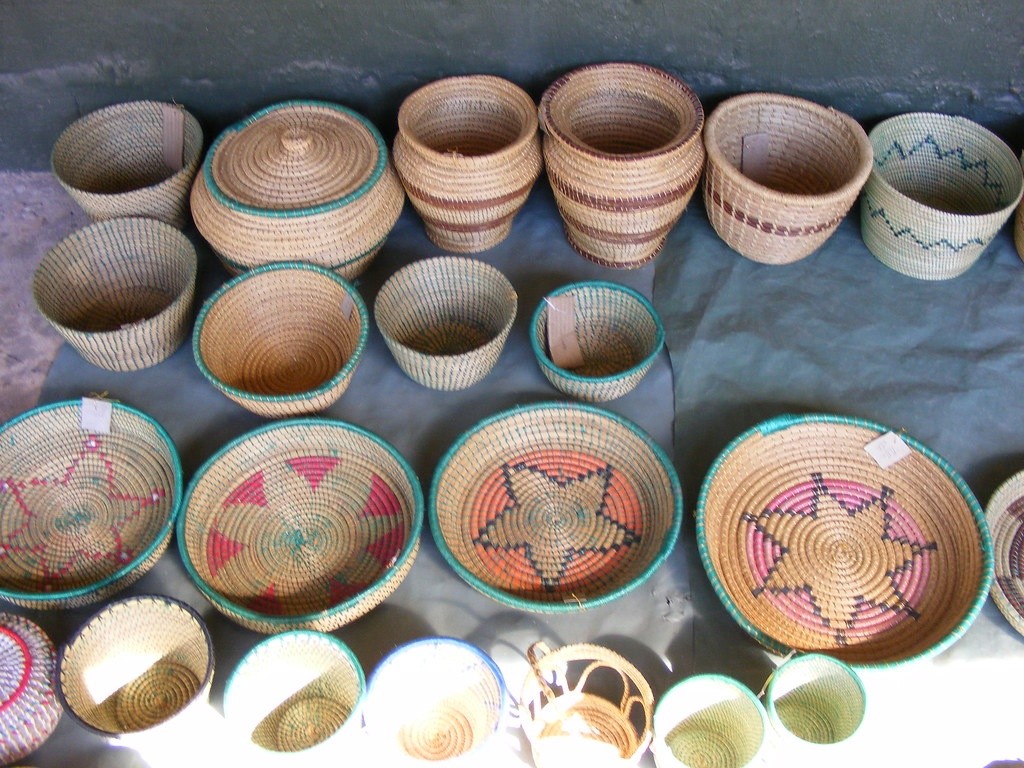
[652,674,767,768]
[373,255,517,391]
[392,74,541,253]
[762,654,866,746]
[56,595,214,738]
[517,642,654,768]
[858,112,1023,280]
[189,101,404,285]
[50,100,204,233]
[32,216,196,373]
[0,399,182,610]
[702,93,872,266]
[363,637,506,761]
[539,62,704,269]
[191,262,368,418]
[984,471,1024,637]
[222,630,365,754]
[530,280,664,402]
[694,413,993,670]
[427,401,682,611]
[177,419,424,634]
[0,613,62,765]
